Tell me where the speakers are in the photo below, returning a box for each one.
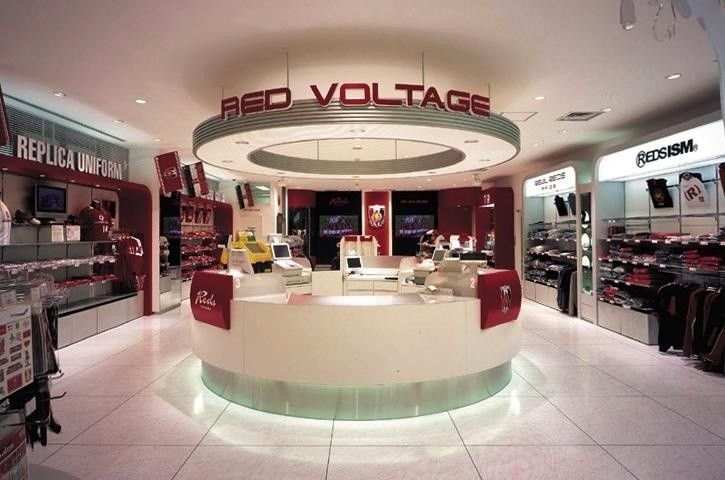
[103,200,116,218]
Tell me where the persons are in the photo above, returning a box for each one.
[77,199,114,242]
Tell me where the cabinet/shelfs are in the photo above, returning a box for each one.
[519,161,725,345]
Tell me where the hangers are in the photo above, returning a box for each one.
[667,272,724,294]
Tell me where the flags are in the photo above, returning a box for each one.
[182,162,210,199]
[154,150,186,196]
[235,184,254,209]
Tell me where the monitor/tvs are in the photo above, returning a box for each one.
[432,249,447,263]
[243,241,266,255]
[346,256,363,271]
[34,183,69,220]
[271,243,292,261]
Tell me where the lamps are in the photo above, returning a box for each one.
[619,0,692,42]
